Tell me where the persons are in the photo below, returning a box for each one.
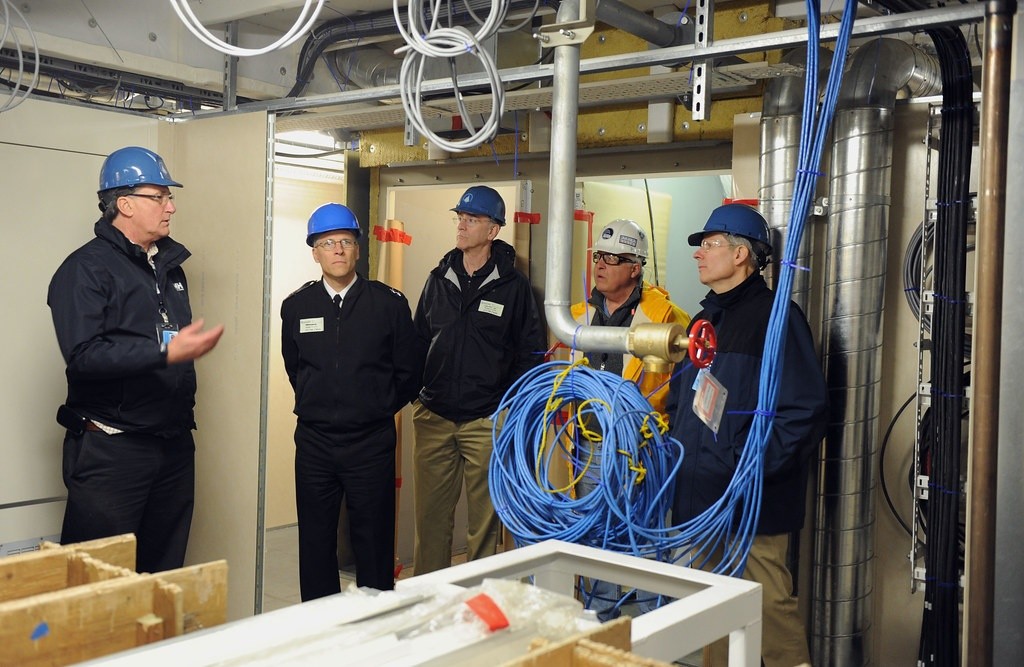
[410,186,549,576]
[47,147,226,574]
[570,220,692,623]
[281,204,412,602]
[666,204,829,667]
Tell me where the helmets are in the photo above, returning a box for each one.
[306,202,360,248]
[97,147,183,192]
[450,186,506,223]
[586,219,651,258]
[688,203,774,257]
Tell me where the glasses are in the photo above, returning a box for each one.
[593,252,646,266]
[701,238,740,253]
[312,239,356,252]
[125,193,177,205]
[452,216,494,224]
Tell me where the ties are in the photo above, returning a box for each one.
[333,294,342,306]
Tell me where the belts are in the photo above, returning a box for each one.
[85,422,102,431]
